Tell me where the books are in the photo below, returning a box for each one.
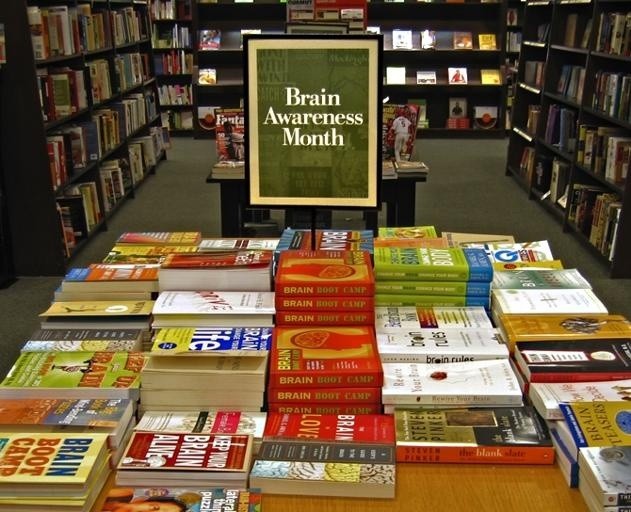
[211,164,245,173]
[447,66,467,84]
[385,66,406,85]
[2,224,631,512]
[381,103,416,161]
[447,118,470,128]
[480,68,500,85]
[197,106,221,133]
[210,172,245,180]
[417,119,429,129]
[415,70,436,83]
[420,29,436,49]
[381,160,428,182]
[197,28,220,50]
[238,27,261,47]
[198,67,216,86]
[478,33,497,49]
[448,97,467,118]
[212,108,245,162]
[392,28,411,48]
[28,0,192,259]
[453,31,471,48]
[505,0,630,224]
[472,106,497,129]
[408,98,427,121]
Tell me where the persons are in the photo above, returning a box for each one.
[389,108,412,159]
